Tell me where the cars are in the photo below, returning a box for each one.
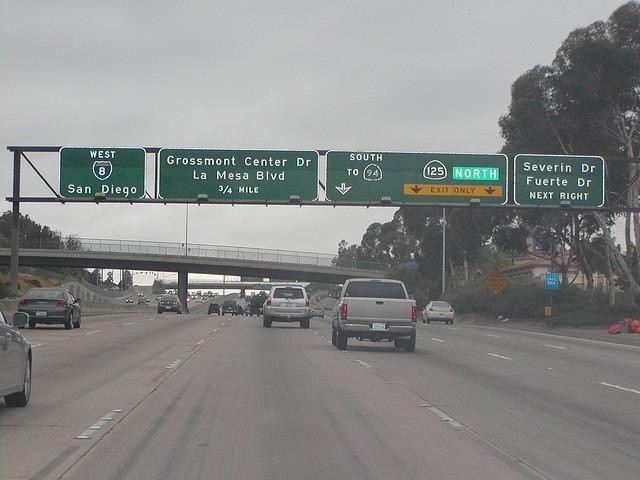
[15,287,83,330]
[0,303,35,411]
[157,293,183,315]
[421,300,455,325]
[120,289,219,304]
[310,306,324,319]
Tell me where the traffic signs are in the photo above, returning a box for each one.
[322,149,510,207]
[544,271,558,290]
[58,146,148,200]
[157,147,320,205]
[512,153,607,208]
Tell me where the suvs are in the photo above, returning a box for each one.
[262,283,311,330]
[222,292,268,318]
[208,303,220,315]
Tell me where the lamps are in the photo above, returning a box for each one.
[559,201,571,208]
[289,194,302,202]
[197,193,209,202]
[381,197,392,205]
[94,193,106,201]
[469,198,481,205]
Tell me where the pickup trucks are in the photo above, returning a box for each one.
[328,277,417,352]
